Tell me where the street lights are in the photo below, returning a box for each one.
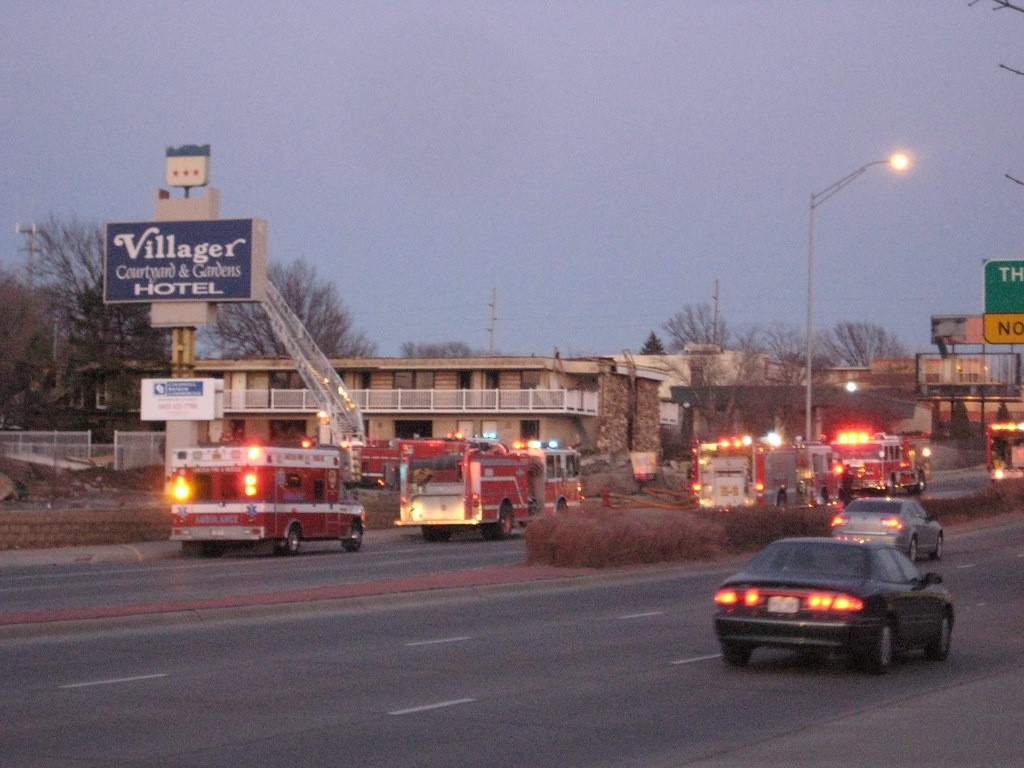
[803,148,913,467]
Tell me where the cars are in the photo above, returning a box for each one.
[713,534,957,674]
[833,498,946,562]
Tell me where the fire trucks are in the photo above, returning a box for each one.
[686,430,831,510]
[820,426,931,499]
[340,431,583,539]
[165,440,366,554]
[985,417,1024,484]
